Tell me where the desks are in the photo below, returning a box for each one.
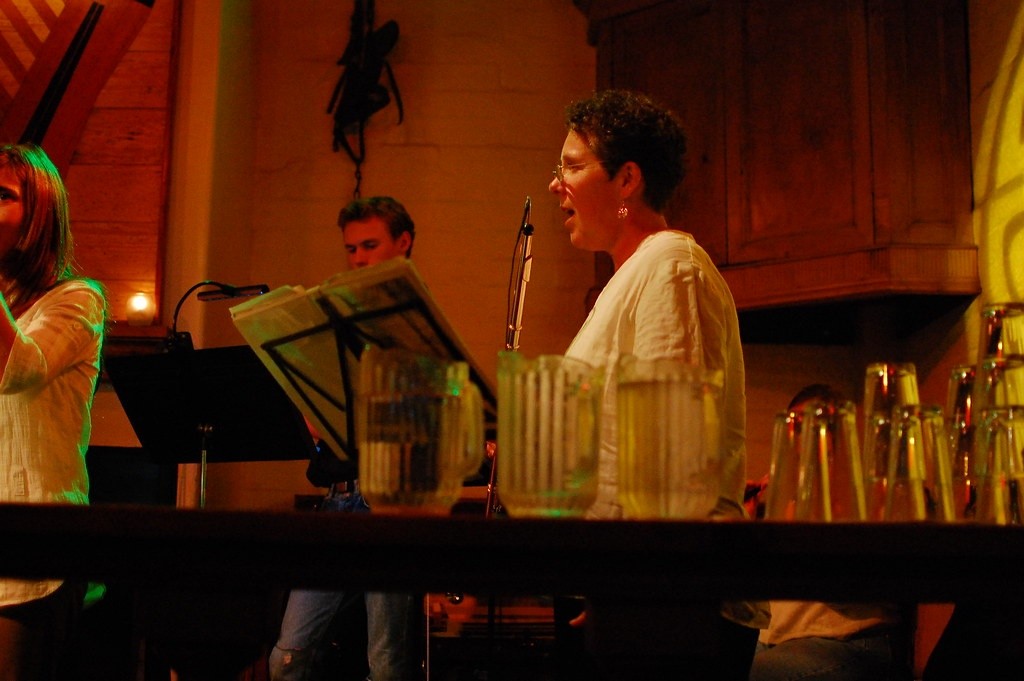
[0,499,1024,681]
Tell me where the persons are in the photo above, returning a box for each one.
[0,141,106,681]
[267,196,424,681]
[748,382,892,680]
[549,90,771,681]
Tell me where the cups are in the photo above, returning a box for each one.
[614,363,725,520]
[764,302,1024,522]
[495,352,611,520]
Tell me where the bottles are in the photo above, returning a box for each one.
[358,348,484,513]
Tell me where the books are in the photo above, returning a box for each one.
[231,258,497,461]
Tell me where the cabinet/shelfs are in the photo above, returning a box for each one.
[576,0,982,308]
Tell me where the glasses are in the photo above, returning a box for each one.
[553,159,628,183]
